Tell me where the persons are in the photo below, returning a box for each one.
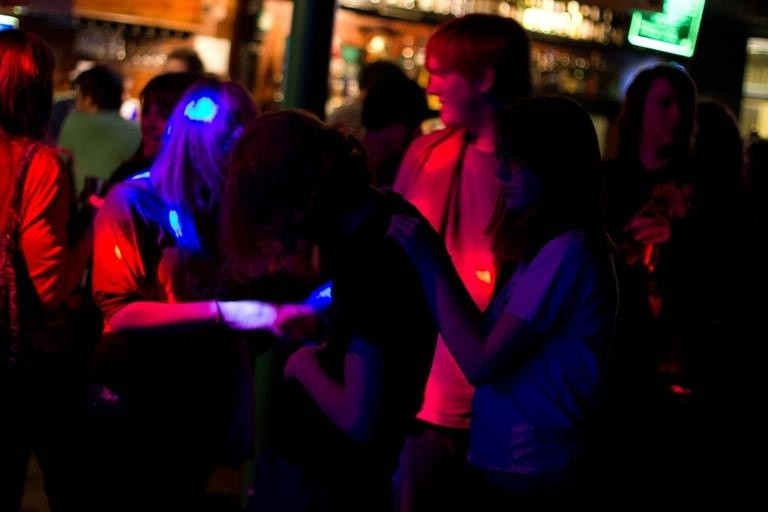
[1,13,768,512]
